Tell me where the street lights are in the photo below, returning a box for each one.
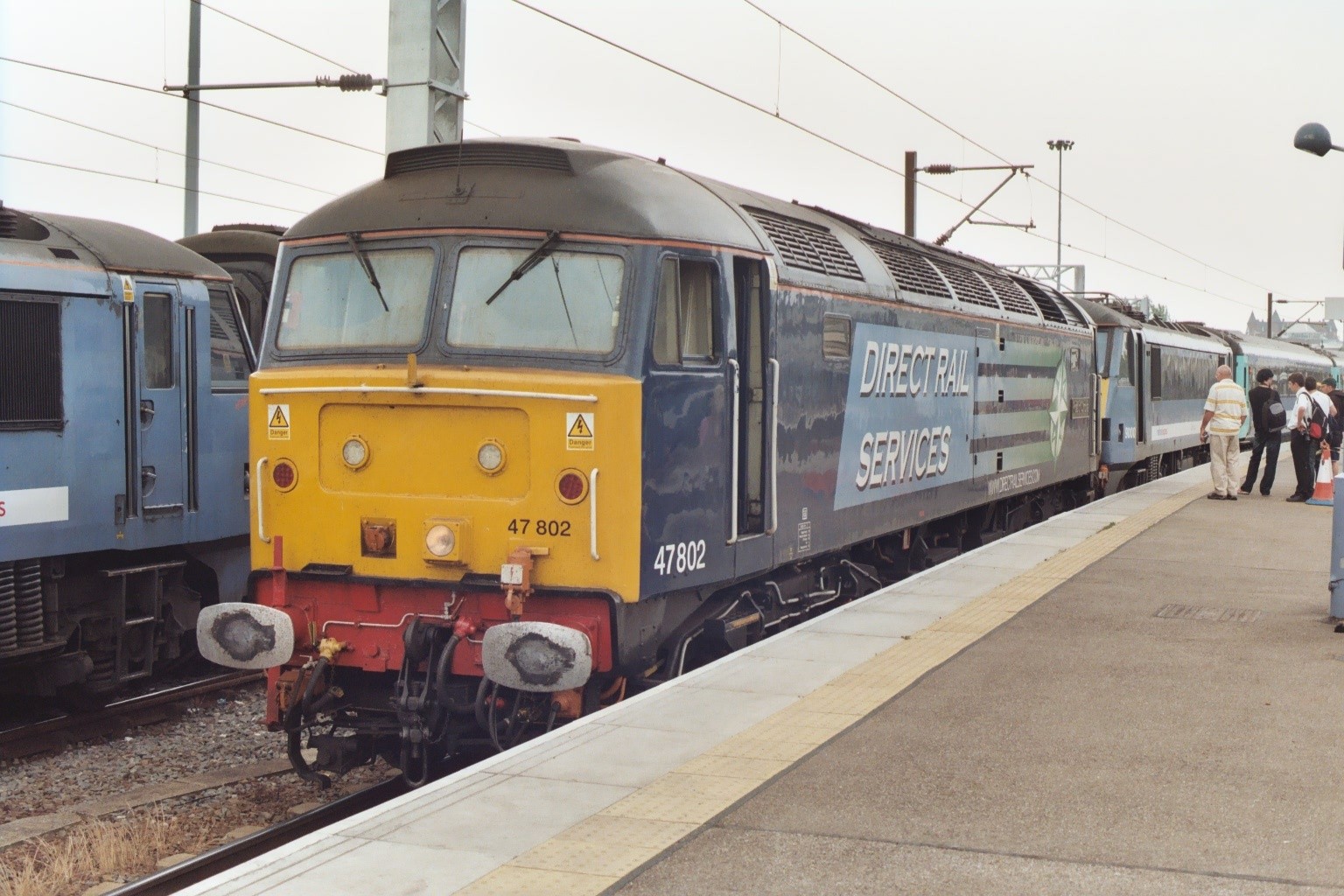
[1046,140,1074,291]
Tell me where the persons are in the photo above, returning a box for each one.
[1305,377,1337,484]
[1321,378,1344,476]
[1200,364,1247,500]
[1237,368,1282,496]
[1286,372,1316,502]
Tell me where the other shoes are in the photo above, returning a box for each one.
[1286,493,1305,502]
[1208,493,1225,500]
[1237,489,1250,495]
[1227,494,1238,500]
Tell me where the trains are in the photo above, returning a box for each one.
[197,136,1344,788]
[0,196,291,725]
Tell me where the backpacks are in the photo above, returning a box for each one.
[1261,389,1287,432]
[1325,392,1344,431]
[1296,392,1328,443]
